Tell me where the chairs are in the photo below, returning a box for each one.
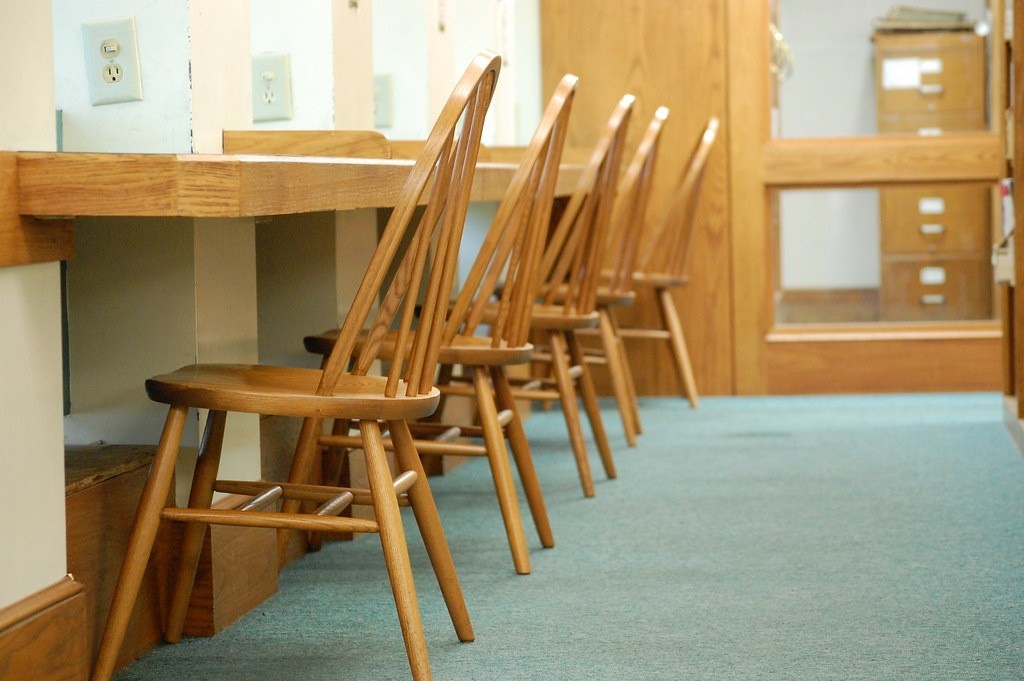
[566,115,721,410]
[90,52,504,681]
[277,73,583,577]
[493,104,673,449]
[415,92,639,499]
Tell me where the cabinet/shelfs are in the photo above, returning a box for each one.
[871,29,991,321]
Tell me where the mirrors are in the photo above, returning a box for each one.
[765,0,1002,323]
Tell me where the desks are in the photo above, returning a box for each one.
[0,131,625,271]
